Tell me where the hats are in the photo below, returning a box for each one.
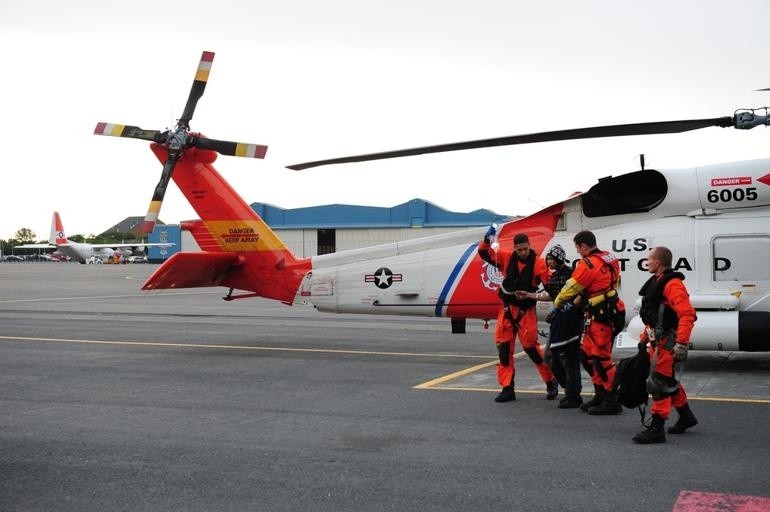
[546,244,566,267]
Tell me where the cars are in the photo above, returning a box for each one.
[1,253,73,263]
[94,253,146,265]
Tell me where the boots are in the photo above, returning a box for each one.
[631,413,667,445]
[667,403,698,434]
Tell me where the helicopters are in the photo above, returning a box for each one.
[89,47,770,364]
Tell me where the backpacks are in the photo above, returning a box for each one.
[614,339,649,408]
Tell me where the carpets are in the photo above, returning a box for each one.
[672,489,770,512]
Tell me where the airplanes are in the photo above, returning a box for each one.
[13,211,177,265]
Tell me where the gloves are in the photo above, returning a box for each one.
[637,340,648,353]
[484,227,496,243]
[670,342,689,362]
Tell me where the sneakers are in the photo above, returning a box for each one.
[580,394,604,412]
[545,376,558,400]
[494,386,516,403]
[588,396,623,415]
[559,393,584,409]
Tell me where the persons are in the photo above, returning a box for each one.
[631,246,698,444]
[478,226,558,403]
[554,230,626,415]
[513,243,586,409]
